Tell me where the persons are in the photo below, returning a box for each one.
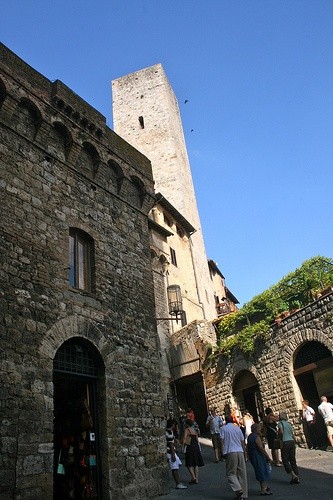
[301,400,319,449]
[220,395,255,463]
[183,419,204,484]
[274,412,300,484]
[206,407,223,462]
[318,396,333,451]
[166,419,187,489]
[219,416,248,500]
[263,408,283,467]
[246,423,273,495]
[176,400,202,446]
[58,427,99,500]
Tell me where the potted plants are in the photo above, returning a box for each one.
[275,285,333,325]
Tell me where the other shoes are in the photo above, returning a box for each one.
[240,497,248,500]
[260,488,273,495]
[215,459,220,463]
[194,480,198,484]
[191,478,196,482]
[290,477,300,484]
[233,490,243,500]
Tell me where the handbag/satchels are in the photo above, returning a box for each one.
[273,438,284,449]
[184,436,192,446]
[57,463,65,474]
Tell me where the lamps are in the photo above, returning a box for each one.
[154,285,184,325]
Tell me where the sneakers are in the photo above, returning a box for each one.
[176,482,187,489]
